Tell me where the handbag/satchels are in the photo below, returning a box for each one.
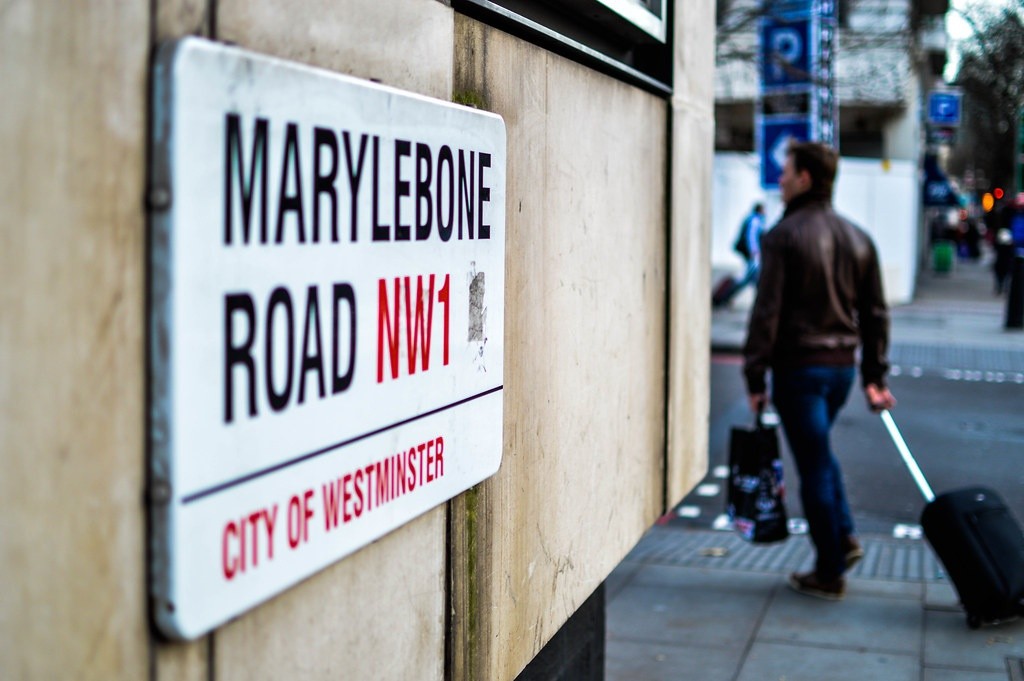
[734,218,753,258]
[724,402,791,545]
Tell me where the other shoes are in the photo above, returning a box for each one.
[837,537,864,574]
[791,569,846,600]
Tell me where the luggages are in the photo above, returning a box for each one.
[711,273,752,309]
[866,383,1024,630]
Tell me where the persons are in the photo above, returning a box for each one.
[742,143,894,601]
[721,203,766,306]
